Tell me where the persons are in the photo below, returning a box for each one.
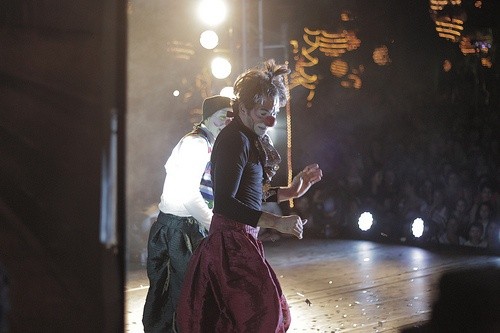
[176,58,323,333]
[142,95,238,333]
[258,150,500,249]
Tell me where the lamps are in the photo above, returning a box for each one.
[410,217,440,250]
